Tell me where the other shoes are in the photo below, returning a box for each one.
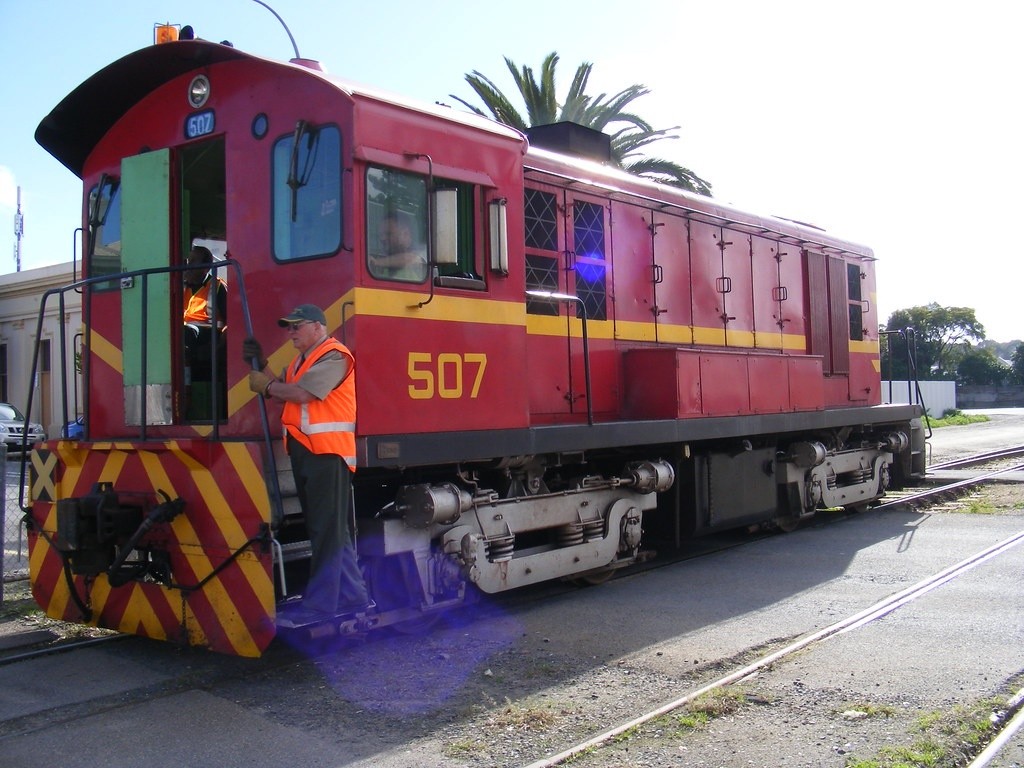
[289,608,335,624]
[337,594,370,612]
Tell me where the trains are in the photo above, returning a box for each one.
[19,22,932,661]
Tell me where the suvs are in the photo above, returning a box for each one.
[0,400,45,455]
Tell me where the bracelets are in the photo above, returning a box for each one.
[263,380,274,399]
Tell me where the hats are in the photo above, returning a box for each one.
[277,304,326,328]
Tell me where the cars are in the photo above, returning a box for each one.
[59,413,91,441]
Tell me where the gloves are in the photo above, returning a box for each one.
[243,335,268,371]
[249,371,275,399]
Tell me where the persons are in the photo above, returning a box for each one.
[369,214,426,279]
[183,246,228,383]
[243,305,368,617]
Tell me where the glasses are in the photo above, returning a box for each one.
[287,322,314,330]
